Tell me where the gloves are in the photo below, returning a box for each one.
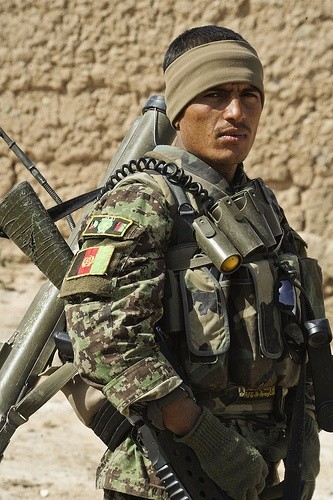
[281,413,320,500]
[173,406,268,500]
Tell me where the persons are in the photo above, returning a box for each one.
[56,23,333,500]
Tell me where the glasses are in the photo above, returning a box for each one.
[274,270,308,352]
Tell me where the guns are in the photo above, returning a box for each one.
[1,179,244,500]
